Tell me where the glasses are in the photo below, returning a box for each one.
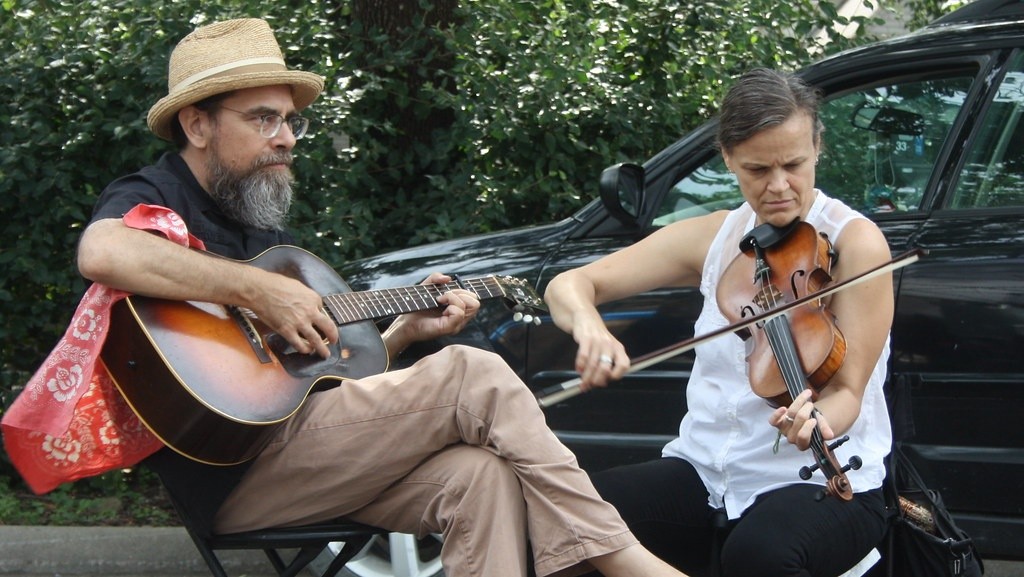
[220,102,310,140]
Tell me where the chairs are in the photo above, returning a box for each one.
[708,372,917,577]
[150,448,394,577]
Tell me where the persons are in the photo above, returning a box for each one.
[77,18,691,577]
[543,66,894,577]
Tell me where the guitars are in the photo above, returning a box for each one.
[715,221,864,506]
[98,234,552,465]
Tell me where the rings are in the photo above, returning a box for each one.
[784,414,794,422]
[600,356,613,364]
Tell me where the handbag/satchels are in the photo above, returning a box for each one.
[883,440,985,577]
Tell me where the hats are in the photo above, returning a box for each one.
[146,18,325,139]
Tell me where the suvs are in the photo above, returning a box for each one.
[327,3,1024,564]
